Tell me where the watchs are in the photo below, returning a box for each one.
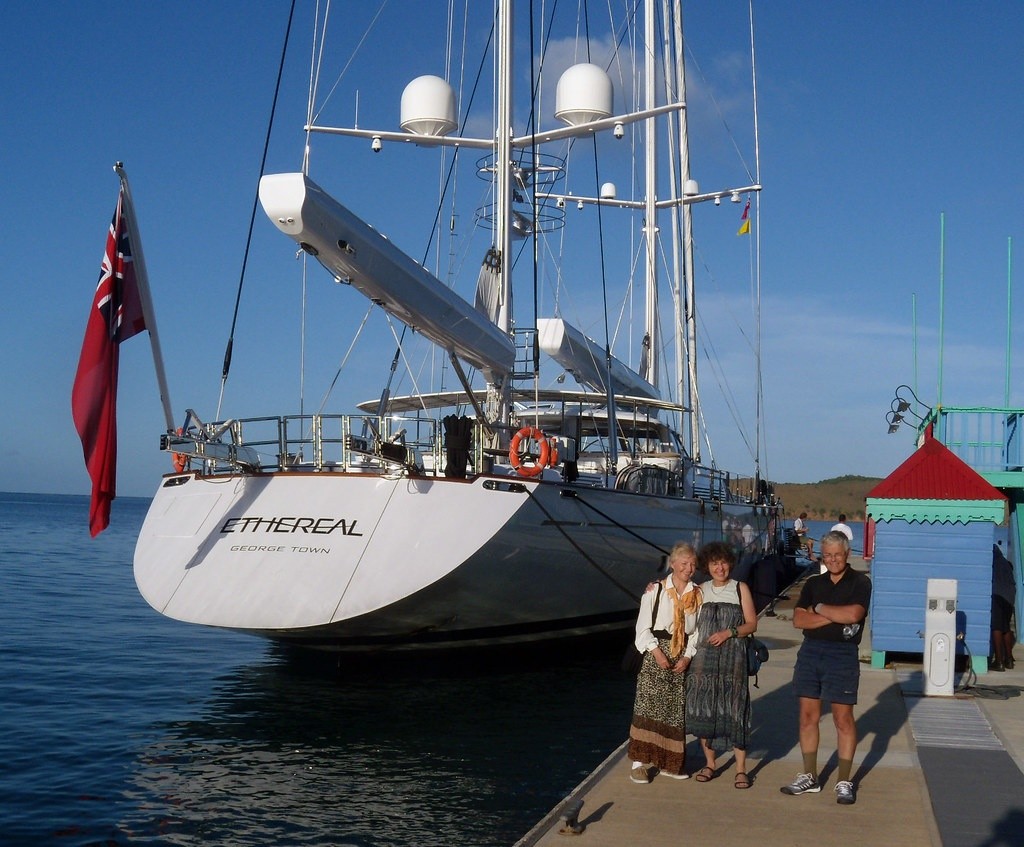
[729,626,739,638]
[812,600,823,615]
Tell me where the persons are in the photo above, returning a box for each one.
[794,512,819,562]
[830,514,854,540]
[781,531,873,805]
[989,544,1016,672]
[626,540,704,785]
[686,540,757,789]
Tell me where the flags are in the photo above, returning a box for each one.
[741,201,750,220]
[736,218,749,236]
[70,182,147,540]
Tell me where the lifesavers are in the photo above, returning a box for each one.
[550,437,560,468]
[509,427,550,476]
[172,427,190,473]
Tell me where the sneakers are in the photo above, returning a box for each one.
[660,768,690,780]
[835,780,855,804]
[780,774,821,795]
[630,766,649,784]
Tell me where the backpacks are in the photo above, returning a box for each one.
[621,640,644,691]
[746,637,769,676]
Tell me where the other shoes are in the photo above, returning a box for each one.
[990,656,1013,672]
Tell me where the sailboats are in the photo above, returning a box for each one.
[132,0,783,656]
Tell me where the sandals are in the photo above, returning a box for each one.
[696,766,714,782]
[734,772,751,789]
